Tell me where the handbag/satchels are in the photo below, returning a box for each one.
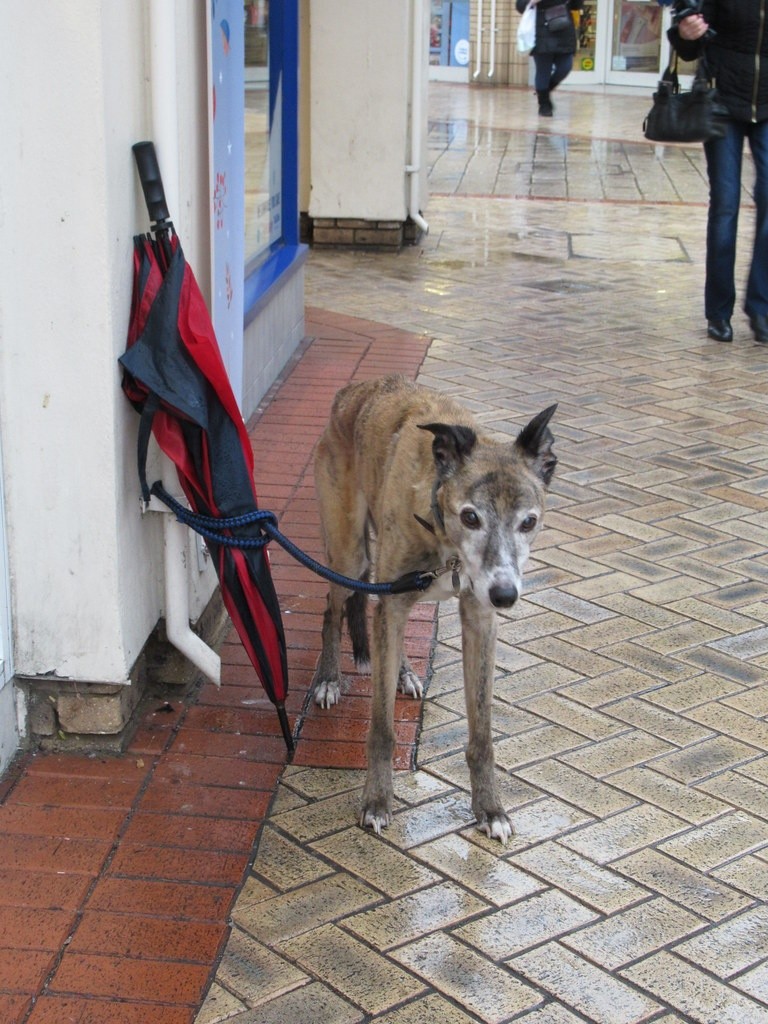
[643,46,726,142]
[516,0,537,55]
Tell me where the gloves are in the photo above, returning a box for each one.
[667,8,717,47]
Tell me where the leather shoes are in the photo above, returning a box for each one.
[749,312,768,342]
[708,319,732,341]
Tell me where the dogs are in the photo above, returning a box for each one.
[308,376,561,850]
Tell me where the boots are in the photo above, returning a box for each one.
[536,88,553,116]
[549,78,557,109]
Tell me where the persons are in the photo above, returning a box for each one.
[518,4,587,115]
[669,1,766,343]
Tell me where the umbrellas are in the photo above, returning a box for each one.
[123,141,298,760]
